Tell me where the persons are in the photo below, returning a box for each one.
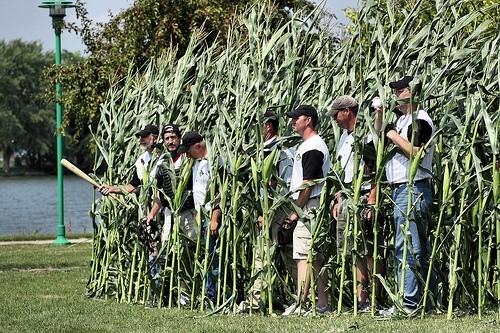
[325,95,380,316]
[98,124,167,308]
[137,124,196,308]
[182,131,246,309]
[239,111,299,313]
[283,104,329,316]
[371,77,434,318]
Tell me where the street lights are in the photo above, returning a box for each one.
[37,0,80,246]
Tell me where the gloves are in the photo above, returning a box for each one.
[384,122,396,139]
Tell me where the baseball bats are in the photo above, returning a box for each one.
[59,157,130,209]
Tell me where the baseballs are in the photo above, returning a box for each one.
[372,98,382,108]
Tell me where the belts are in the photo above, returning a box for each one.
[360,190,370,195]
[390,178,427,189]
[294,196,320,201]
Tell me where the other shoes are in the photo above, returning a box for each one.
[354,302,375,312]
[144,301,164,309]
[374,306,417,318]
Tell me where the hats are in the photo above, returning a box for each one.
[244,112,278,122]
[389,76,422,89]
[161,124,182,138]
[286,105,318,121]
[182,132,203,153]
[325,95,358,116]
[151,143,164,151]
[135,125,159,138]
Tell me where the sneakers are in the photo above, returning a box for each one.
[282,302,307,316]
[302,304,332,317]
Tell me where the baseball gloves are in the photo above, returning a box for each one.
[274,217,298,246]
[358,205,382,233]
